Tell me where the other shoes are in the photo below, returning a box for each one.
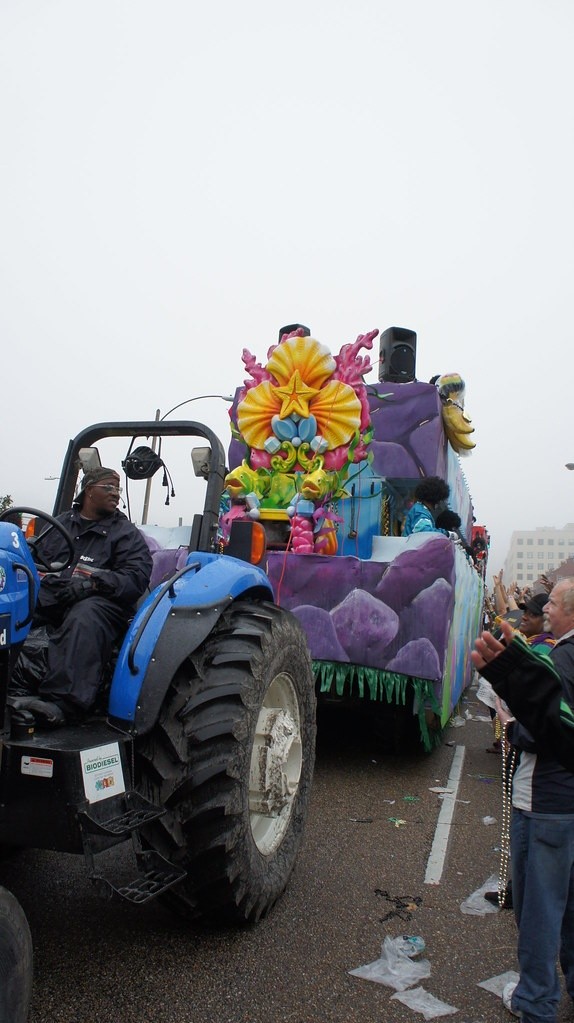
[28,700,66,725]
[486,748,502,753]
[502,982,522,1017]
[485,879,513,909]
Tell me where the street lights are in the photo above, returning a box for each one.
[142,395,235,525]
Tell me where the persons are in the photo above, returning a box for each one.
[401,477,459,539]
[472,566,574,1023]
[434,509,462,534]
[11,468,156,729]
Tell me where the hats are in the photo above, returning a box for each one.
[518,593,549,615]
[74,467,120,503]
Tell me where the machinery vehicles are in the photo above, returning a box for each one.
[0,422,317,1023]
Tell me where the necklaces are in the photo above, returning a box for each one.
[495,716,515,908]
[495,715,501,739]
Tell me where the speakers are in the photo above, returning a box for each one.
[278,324,310,344]
[379,326,417,384]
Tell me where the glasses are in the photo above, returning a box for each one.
[89,485,122,494]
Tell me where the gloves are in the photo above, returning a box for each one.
[48,577,93,606]
